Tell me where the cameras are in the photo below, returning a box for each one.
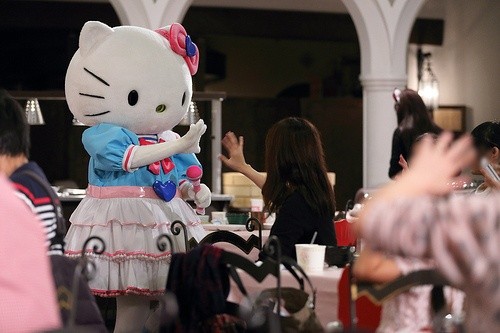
[476,154,500,188]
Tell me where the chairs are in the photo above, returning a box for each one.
[48,217,451,333]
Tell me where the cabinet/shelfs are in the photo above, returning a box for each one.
[5,89,235,231]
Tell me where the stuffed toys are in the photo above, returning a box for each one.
[62,19,208,333]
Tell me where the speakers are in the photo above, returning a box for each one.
[409,17,445,46]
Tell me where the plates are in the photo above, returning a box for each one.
[203,223,246,231]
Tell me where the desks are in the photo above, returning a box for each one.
[227,264,343,333]
[200,224,272,265]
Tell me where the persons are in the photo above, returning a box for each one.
[344,85,500,333]
[177,115,343,333]
[0,85,69,332]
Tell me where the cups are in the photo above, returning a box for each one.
[24,98,45,125]
[211,211,226,224]
[179,101,200,125]
[73,116,91,126]
[251,198,264,229]
[296,243,327,275]
